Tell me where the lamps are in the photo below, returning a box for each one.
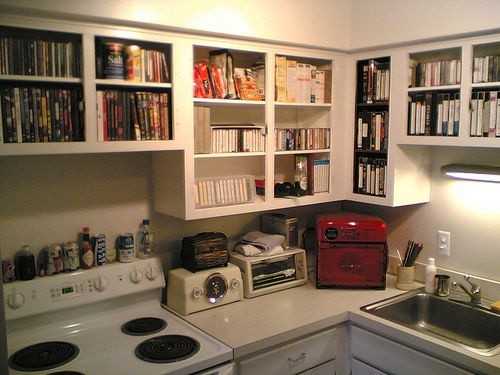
[442,166,499,184]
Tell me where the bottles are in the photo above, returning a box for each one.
[2,257,13,283]
[136,219,153,259]
[80,227,95,269]
[125,44,142,82]
[44,243,63,276]
[14,244,36,280]
[102,42,126,80]
[118,232,136,263]
[96,233,107,265]
[63,241,80,271]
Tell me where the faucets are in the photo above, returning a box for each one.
[452,274,481,303]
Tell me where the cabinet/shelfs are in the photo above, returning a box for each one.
[342,327,476,375]
[237,324,350,373]
[185,32,344,222]
[343,47,432,206]
[1,15,183,156]
[396,34,499,147]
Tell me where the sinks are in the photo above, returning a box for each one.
[372,295,500,351]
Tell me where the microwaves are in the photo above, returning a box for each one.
[229,246,309,299]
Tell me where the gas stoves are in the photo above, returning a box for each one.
[1,256,236,375]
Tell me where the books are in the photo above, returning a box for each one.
[3,87,83,143]
[141,49,169,83]
[409,60,461,87]
[97,41,104,79]
[0,37,80,78]
[295,155,329,196]
[357,110,389,152]
[96,90,170,141]
[275,56,331,104]
[274,128,331,151]
[472,55,500,83]
[358,157,387,197]
[408,93,460,136]
[471,91,500,138]
[357,58,390,105]
[195,105,265,154]
[194,178,252,209]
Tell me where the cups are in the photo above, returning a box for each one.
[396,262,415,292]
[433,274,452,297]
